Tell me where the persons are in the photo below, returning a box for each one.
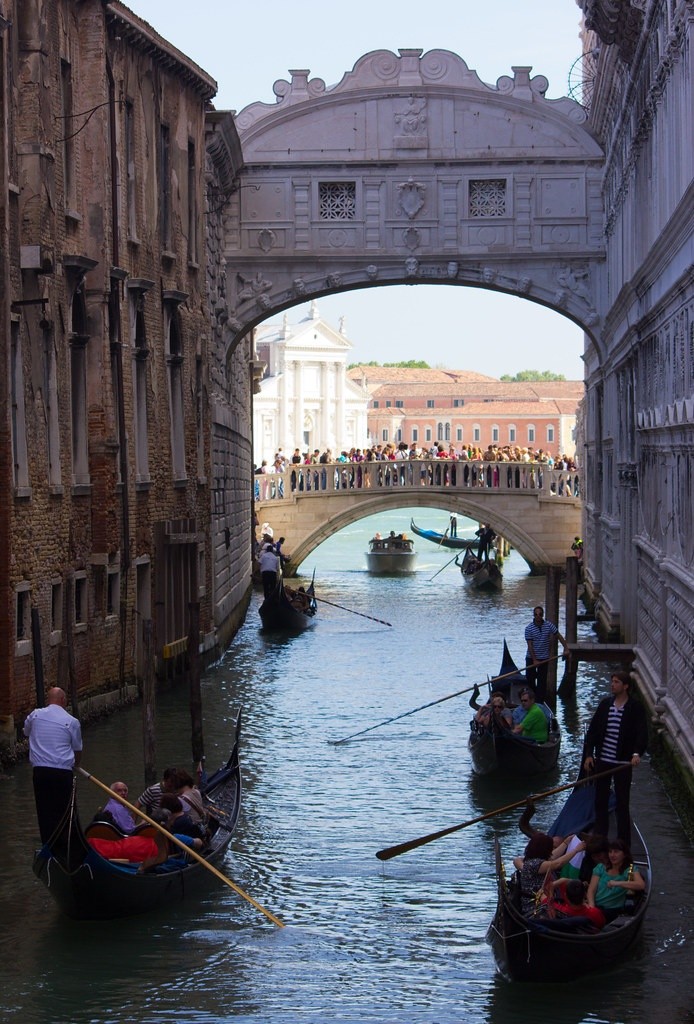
[449,510,458,537]
[525,606,570,704]
[238,273,272,300]
[513,832,646,927]
[284,584,309,614]
[583,671,649,859]
[475,522,493,564]
[251,439,581,502]
[372,531,409,550]
[572,535,583,565]
[23,687,84,850]
[555,268,596,309]
[470,684,548,745]
[96,767,205,849]
[253,511,290,599]
[394,96,427,137]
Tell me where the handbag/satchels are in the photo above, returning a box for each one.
[627,864,635,895]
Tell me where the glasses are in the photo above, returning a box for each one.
[534,612,543,617]
[493,705,503,709]
[521,699,528,703]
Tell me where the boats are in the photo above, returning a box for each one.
[32,705,243,925]
[411,517,494,549]
[366,531,416,574]
[258,568,318,636]
[485,723,652,987]
[455,542,503,593]
[467,637,561,778]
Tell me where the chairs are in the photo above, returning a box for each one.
[85,820,165,839]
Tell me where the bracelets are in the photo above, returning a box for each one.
[633,748,640,757]
[563,840,568,846]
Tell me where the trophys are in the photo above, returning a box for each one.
[625,864,636,906]
[531,889,545,920]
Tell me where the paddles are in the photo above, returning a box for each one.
[284,586,392,627]
[374,762,633,861]
[72,765,285,929]
[438,518,453,549]
[333,655,562,744]
[429,535,479,581]
